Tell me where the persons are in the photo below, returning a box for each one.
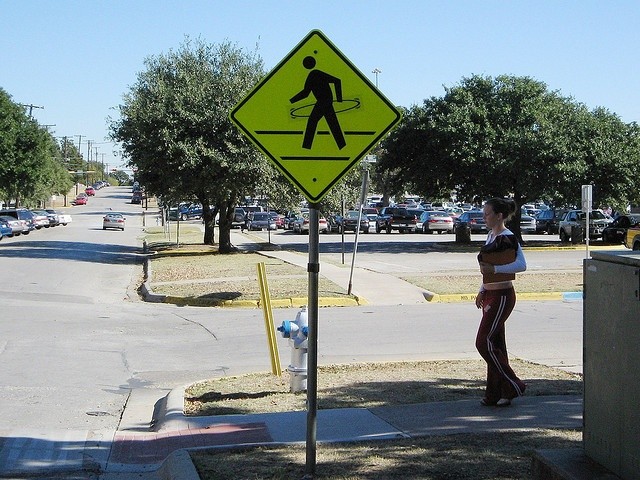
[476,198,527,408]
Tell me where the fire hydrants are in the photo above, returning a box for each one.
[278,306,309,395]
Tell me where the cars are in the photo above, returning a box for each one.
[558,209,608,241]
[0,207,72,237]
[331,210,370,233]
[75,180,110,205]
[247,212,277,231]
[284,207,328,234]
[103,213,126,231]
[375,206,416,234]
[417,211,454,233]
[459,211,490,233]
[177,202,204,221]
[521,203,565,234]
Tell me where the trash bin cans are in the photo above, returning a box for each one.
[455,222,470,244]
[572,225,584,243]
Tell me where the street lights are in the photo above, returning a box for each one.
[370,68,382,91]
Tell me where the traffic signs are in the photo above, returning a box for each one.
[582,185,592,212]
[229,29,403,205]
